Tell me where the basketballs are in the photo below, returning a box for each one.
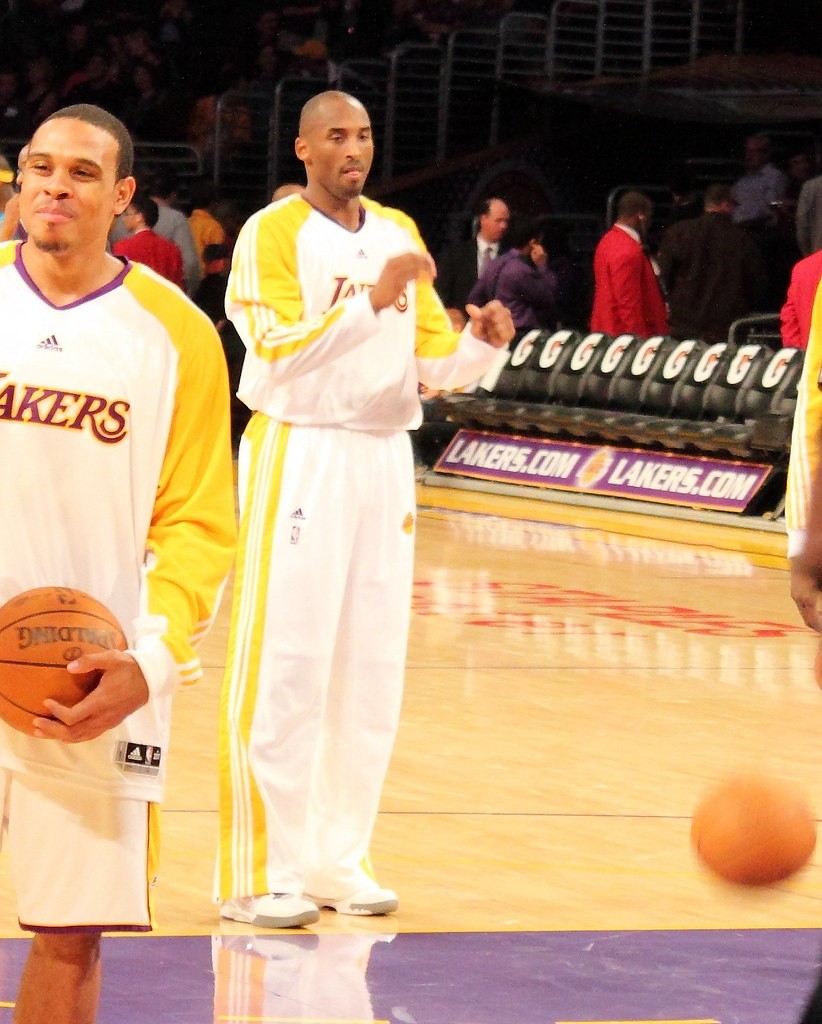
[687,774,817,884]
[0,587,128,738]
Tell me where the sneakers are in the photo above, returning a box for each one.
[302,885,399,916]
[219,892,322,927]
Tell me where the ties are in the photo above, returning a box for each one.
[483,246,492,280]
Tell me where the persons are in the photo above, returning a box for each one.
[708,126,822,311]
[433,197,510,328]
[271,183,305,204]
[0,0,515,232]
[0,143,27,266]
[591,191,671,341]
[104,176,234,327]
[211,933,398,1024]
[212,90,515,927]
[785,277,822,682]
[658,183,769,348]
[780,250,822,351]
[465,216,556,352]
[0,104,234,1024]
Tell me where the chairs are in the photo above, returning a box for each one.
[447,328,807,461]
